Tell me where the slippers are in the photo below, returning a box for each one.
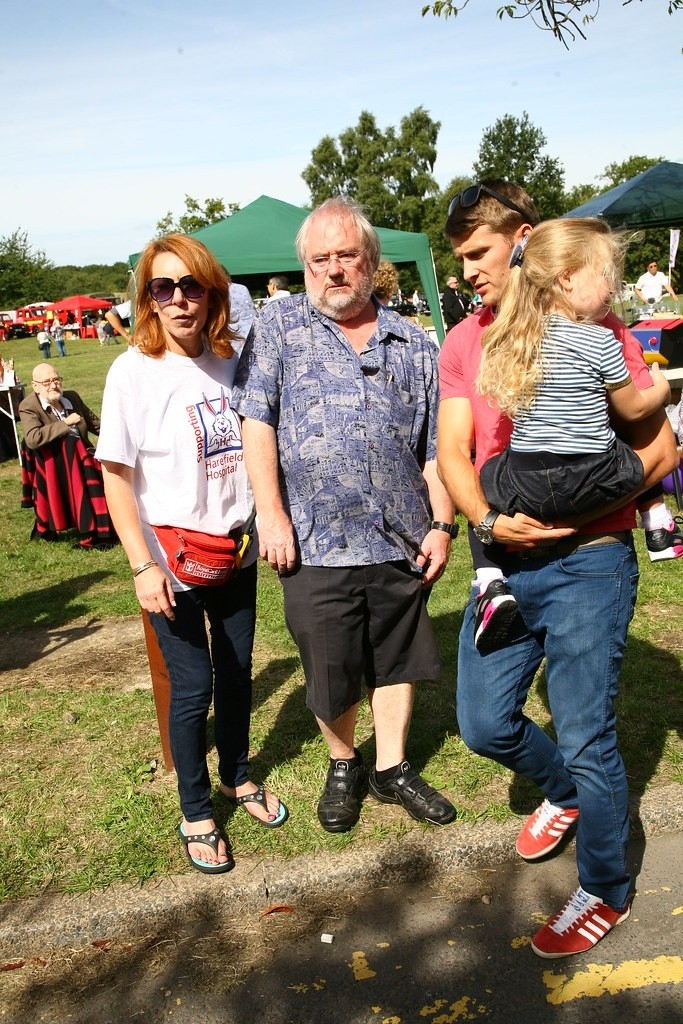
[218,784,288,827]
[178,821,232,873]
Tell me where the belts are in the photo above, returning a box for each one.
[522,532,627,557]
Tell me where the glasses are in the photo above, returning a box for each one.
[32,377,63,386]
[448,184,531,224]
[650,265,656,268]
[305,249,365,267]
[146,274,204,301]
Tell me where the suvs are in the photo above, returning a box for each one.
[0,314,26,340]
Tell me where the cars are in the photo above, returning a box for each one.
[388,293,473,316]
[16,306,49,335]
[626,283,635,300]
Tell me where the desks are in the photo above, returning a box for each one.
[62,326,118,339]
[0,383,27,467]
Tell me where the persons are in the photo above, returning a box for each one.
[231,195,456,832]
[94,234,289,876]
[95,264,258,358]
[19,363,101,450]
[266,276,290,302]
[440,275,469,333]
[634,258,679,308]
[412,290,421,317]
[435,178,683,961]
[37,315,67,359]
[0,319,7,344]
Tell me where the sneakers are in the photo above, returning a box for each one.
[516,799,579,859]
[318,747,367,831]
[531,885,630,958]
[460,580,518,650]
[365,764,456,825]
[645,516,683,562]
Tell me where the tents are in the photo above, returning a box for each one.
[128,195,445,348]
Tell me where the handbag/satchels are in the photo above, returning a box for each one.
[38,344,44,350]
[152,525,234,586]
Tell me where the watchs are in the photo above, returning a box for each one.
[473,510,502,545]
[431,521,459,539]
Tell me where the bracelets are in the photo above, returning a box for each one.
[132,559,157,577]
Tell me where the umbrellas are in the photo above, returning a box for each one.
[46,295,112,334]
